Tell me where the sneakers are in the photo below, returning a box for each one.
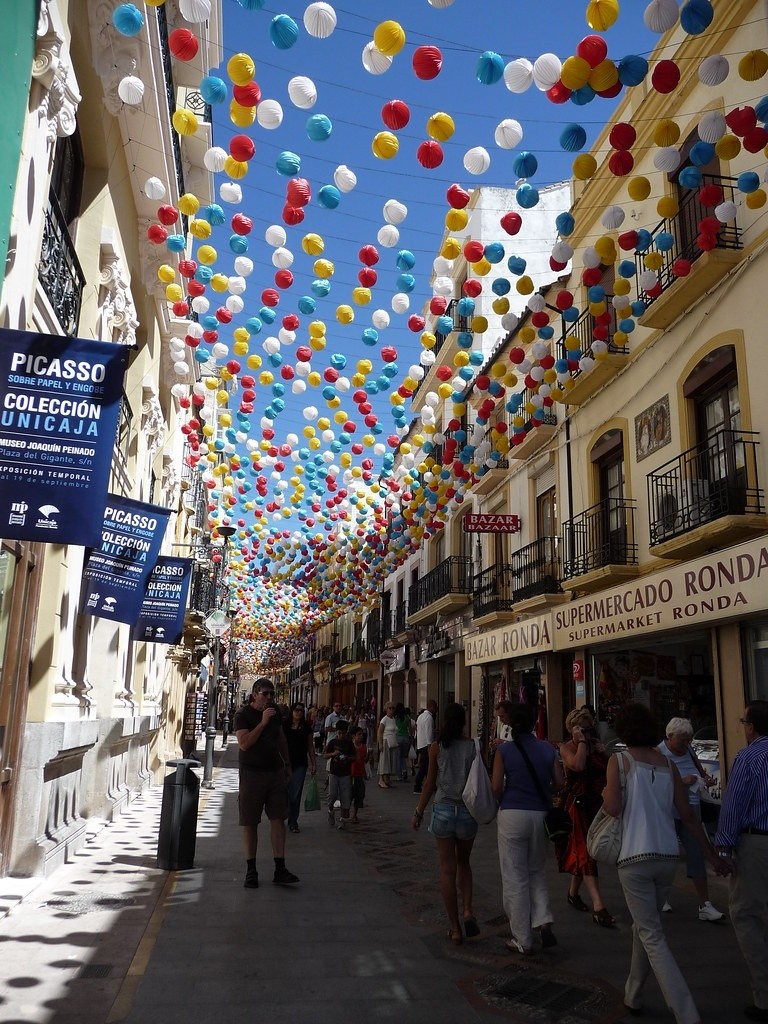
[662,901,672,912]
[698,900,727,920]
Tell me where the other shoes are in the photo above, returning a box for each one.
[289,825,300,833]
[328,810,345,831]
[592,907,616,928]
[624,1002,642,1016]
[352,816,360,824]
[567,889,587,912]
[244,870,260,888]
[449,917,480,946]
[271,870,301,886]
[378,767,423,795]
[744,1005,768,1024]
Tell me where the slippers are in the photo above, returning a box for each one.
[504,938,532,954]
[540,926,557,947]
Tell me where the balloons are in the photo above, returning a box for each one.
[494,118,524,150]
[269,13,299,50]
[288,76,317,109]
[168,28,199,62]
[426,111,455,142]
[303,2,338,38]
[306,114,332,141]
[413,45,442,80]
[381,100,411,131]
[112,0,768,680]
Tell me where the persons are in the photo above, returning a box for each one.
[652,716,726,921]
[220,694,254,733]
[601,704,730,1024]
[282,702,317,833]
[492,704,565,953]
[712,699,768,1024]
[276,703,290,723]
[559,705,617,926]
[309,696,442,831]
[233,679,299,889]
[489,700,538,791]
[410,703,496,940]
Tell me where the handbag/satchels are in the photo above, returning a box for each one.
[545,809,573,840]
[304,776,321,811]
[586,752,635,865]
[408,744,417,760]
[461,738,498,826]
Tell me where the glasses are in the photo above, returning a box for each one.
[740,718,752,724]
[258,691,274,697]
[295,708,304,712]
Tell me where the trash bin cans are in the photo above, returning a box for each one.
[156,759,203,870]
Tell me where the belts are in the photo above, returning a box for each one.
[739,826,768,836]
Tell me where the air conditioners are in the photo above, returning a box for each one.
[655,480,712,539]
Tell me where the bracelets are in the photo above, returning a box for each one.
[718,850,730,858]
[413,809,421,818]
[578,739,586,743]
